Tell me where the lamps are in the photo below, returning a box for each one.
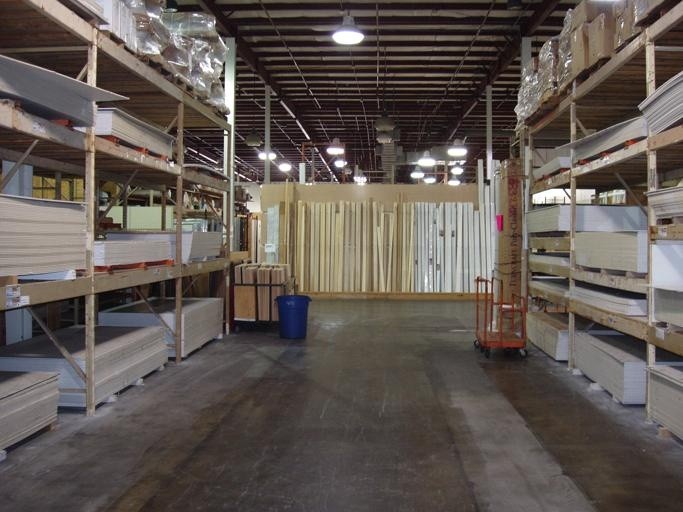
[332,9,365,46]
[266,102,473,187]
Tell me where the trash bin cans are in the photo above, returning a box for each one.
[275,295,312,339]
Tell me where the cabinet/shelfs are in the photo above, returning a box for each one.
[486,1,683,443]
[0,1,262,511]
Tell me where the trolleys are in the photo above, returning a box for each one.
[474,275,529,359]
[232,275,296,335]
